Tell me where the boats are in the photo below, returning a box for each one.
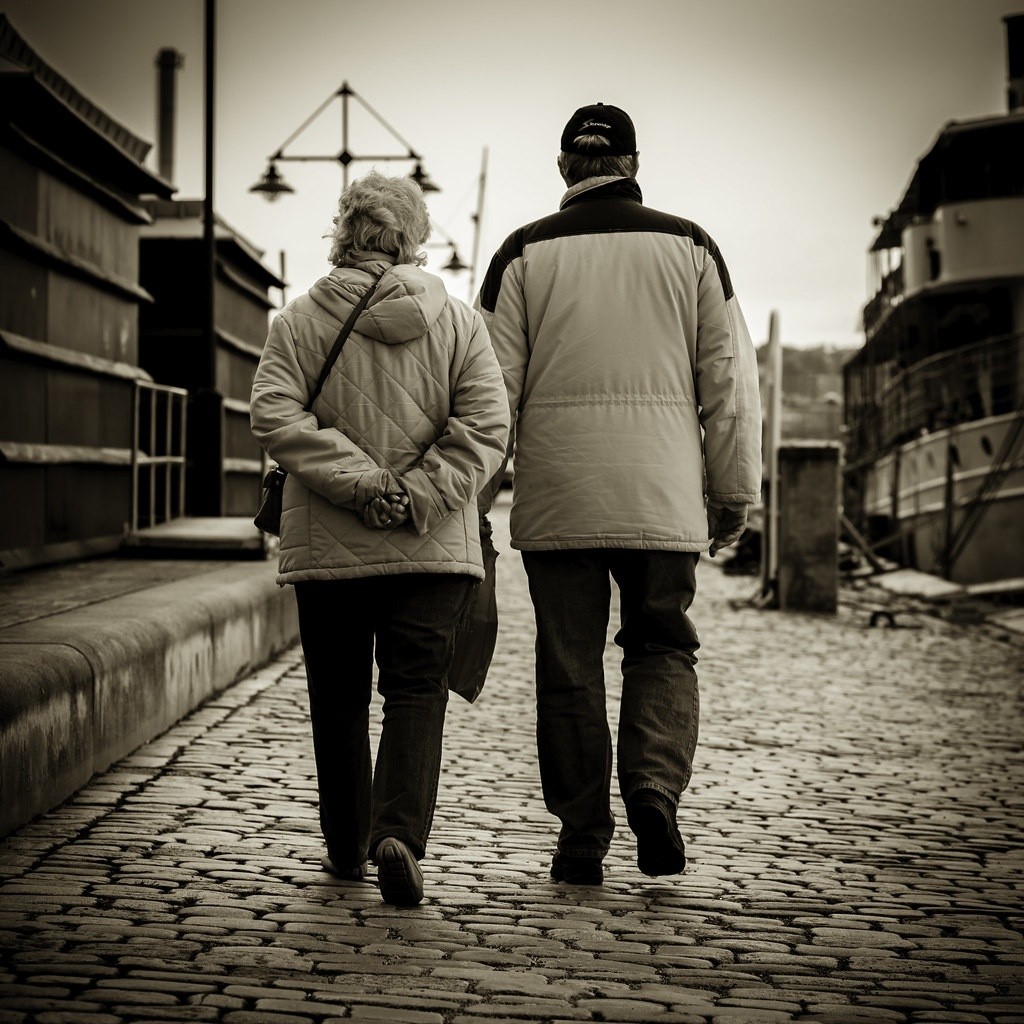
[841,9,1024,587]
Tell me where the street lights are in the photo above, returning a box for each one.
[422,238,470,275]
[247,80,441,218]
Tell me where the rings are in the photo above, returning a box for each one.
[384,518,391,525]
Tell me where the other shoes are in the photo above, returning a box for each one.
[376,837,424,907]
[322,853,368,882]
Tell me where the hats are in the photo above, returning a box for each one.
[560,102,638,157]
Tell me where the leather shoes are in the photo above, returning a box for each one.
[626,788,687,878]
[550,851,604,886]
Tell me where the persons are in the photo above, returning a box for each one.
[470,101,761,885]
[249,173,510,908]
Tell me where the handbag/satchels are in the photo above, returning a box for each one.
[254,465,287,537]
[447,515,499,704]
